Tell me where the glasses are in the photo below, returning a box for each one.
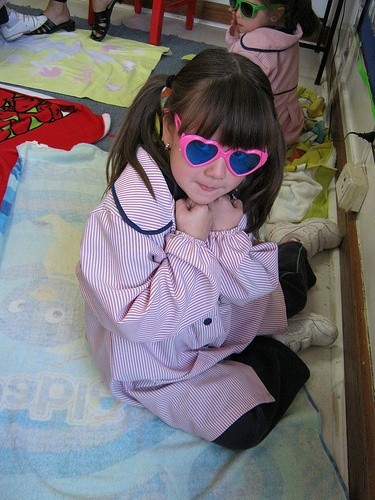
[229,0,284,18]
[162,107,269,178]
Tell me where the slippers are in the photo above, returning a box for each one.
[89,0,117,42]
[23,12,76,34]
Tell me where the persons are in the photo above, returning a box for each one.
[225,0,324,151]
[75,48,342,453]
[0,0,116,42]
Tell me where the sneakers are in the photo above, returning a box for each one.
[265,217,343,261]
[1,8,48,42]
[271,309,339,354]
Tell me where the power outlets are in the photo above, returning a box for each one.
[336,163,369,212]
